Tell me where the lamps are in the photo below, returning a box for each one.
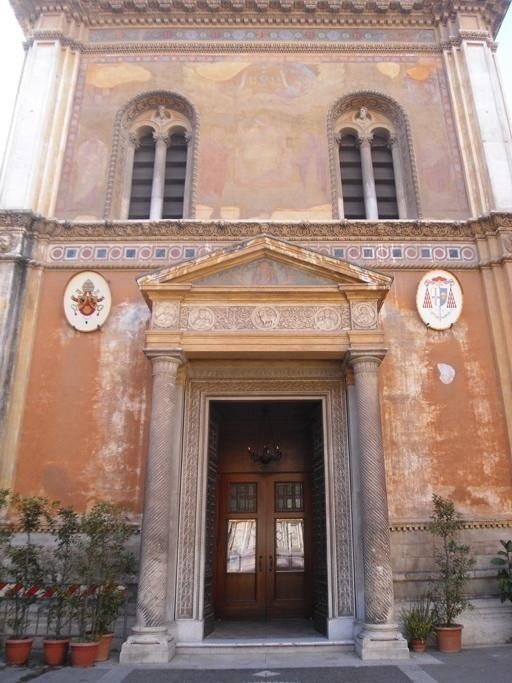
[247,407,281,464]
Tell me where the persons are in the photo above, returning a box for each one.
[355,106,370,124]
[154,105,167,123]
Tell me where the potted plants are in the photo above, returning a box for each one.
[0,487,142,664]
[401,593,438,652]
[423,487,475,652]
